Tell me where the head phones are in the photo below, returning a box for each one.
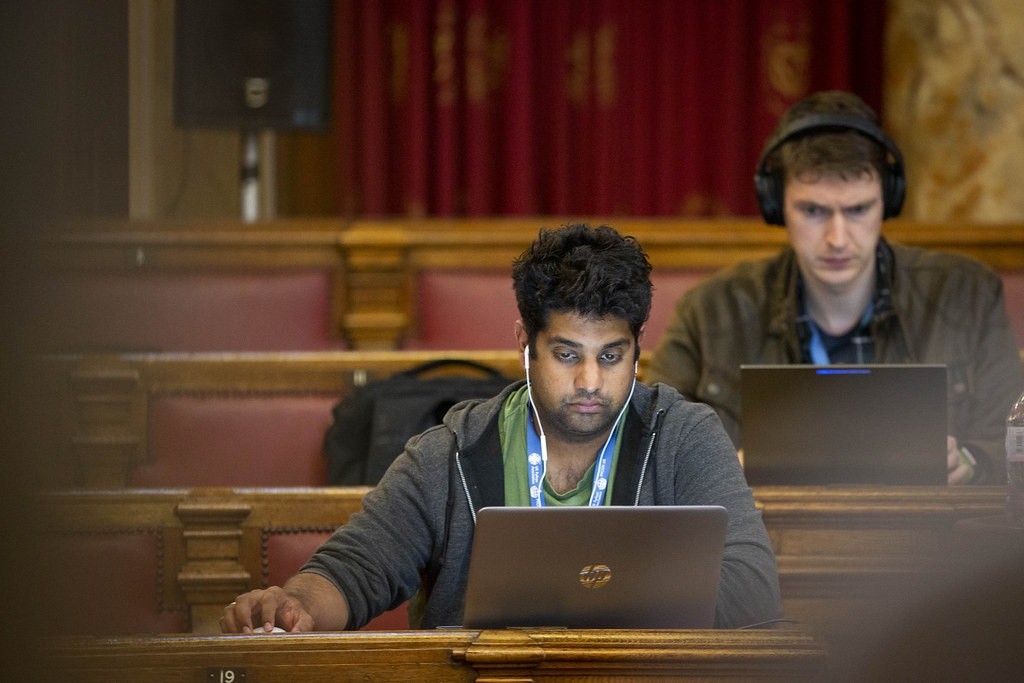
[751,112,905,227]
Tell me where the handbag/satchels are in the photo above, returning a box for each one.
[318,356,520,488]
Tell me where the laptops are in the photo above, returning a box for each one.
[739,361,949,490]
[460,504,726,629]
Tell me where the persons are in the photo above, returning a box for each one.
[218,225,782,635]
[640,91,1024,486]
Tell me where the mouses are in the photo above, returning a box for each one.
[254,624,287,635]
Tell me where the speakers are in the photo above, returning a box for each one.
[172,0,337,135]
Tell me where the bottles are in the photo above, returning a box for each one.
[238,79,276,221]
[1004,392,1024,512]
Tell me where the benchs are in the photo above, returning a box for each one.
[0,218,1024,683]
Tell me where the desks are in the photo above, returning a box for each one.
[0,625,835,683]
[17,350,661,396]
[29,488,1006,532]
[0,218,1024,275]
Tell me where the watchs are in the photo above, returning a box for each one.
[958,442,985,485]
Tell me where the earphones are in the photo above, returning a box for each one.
[523,343,531,372]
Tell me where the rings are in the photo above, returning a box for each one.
[231,602,236,605]
